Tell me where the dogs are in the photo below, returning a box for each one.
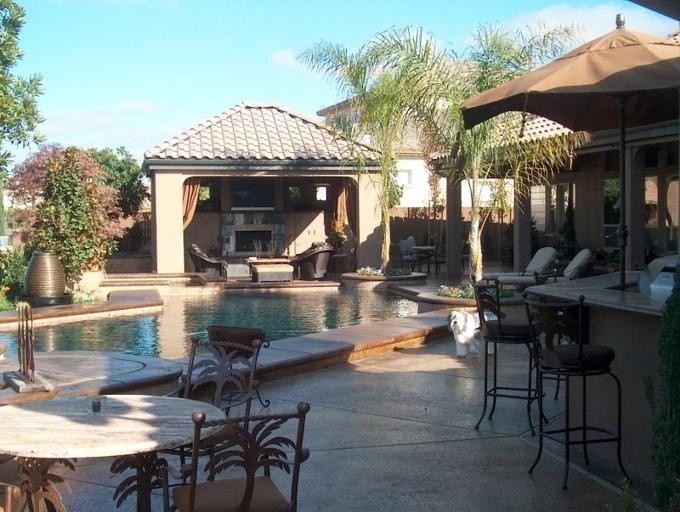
[448,310,498,358]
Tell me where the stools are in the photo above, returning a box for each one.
[251,263,295,283]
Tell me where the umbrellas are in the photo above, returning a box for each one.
[460,13,680,291]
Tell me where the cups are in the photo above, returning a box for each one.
[85,395,106,415]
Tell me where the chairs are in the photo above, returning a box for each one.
[497,246,592,283]
[473,274,549,436]
[291,240,335,281]
[482,245,557,284]
[522,295,632,490]
[173,401,310,512]
[141,334,263,509]
[189,243,229,281]
[397,235,417,272]
[433,243,447,275]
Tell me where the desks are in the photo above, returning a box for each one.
[245,257,291,278]
[411,245,437,273]
[1,394,227,511]
